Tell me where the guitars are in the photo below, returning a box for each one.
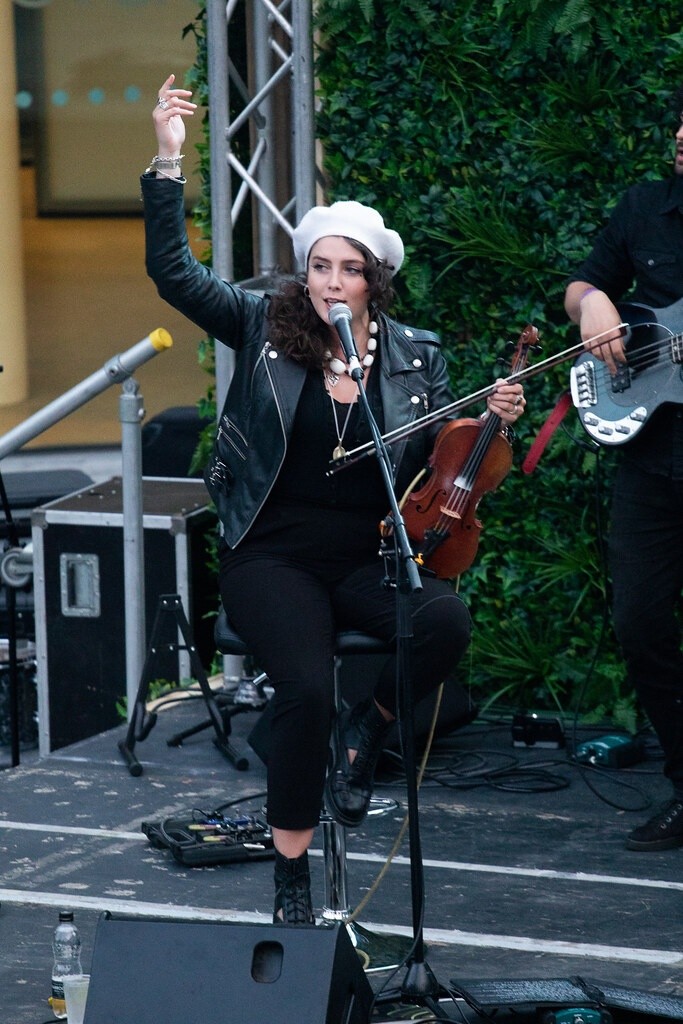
[567,295,683,448]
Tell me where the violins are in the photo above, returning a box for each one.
[382,323,546,579]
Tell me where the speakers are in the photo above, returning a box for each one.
[82,910,374,1024]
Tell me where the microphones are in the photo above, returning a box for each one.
[327,303,364,382]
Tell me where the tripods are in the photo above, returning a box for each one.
[354,379,472,1024]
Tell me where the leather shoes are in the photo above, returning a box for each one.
[323,695,400,828]
[273,845,316,925]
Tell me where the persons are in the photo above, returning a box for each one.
[564,112,683,850]
[140,74,526,924]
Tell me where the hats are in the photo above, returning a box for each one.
[292,201,405,288]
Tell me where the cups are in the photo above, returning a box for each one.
[63,975,89,1024]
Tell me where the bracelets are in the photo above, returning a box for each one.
[576,288,599,311]
[140,155,187,201]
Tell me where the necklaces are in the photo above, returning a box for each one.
[323,315,378,460]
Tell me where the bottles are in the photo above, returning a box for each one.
[51,910,83,1019]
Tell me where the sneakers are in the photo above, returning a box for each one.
[623,798,683,852]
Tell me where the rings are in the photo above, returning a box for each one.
[157,97,166,104]
[513,396,522,405]
[508,405,519,416]
[160,102,169,110]
